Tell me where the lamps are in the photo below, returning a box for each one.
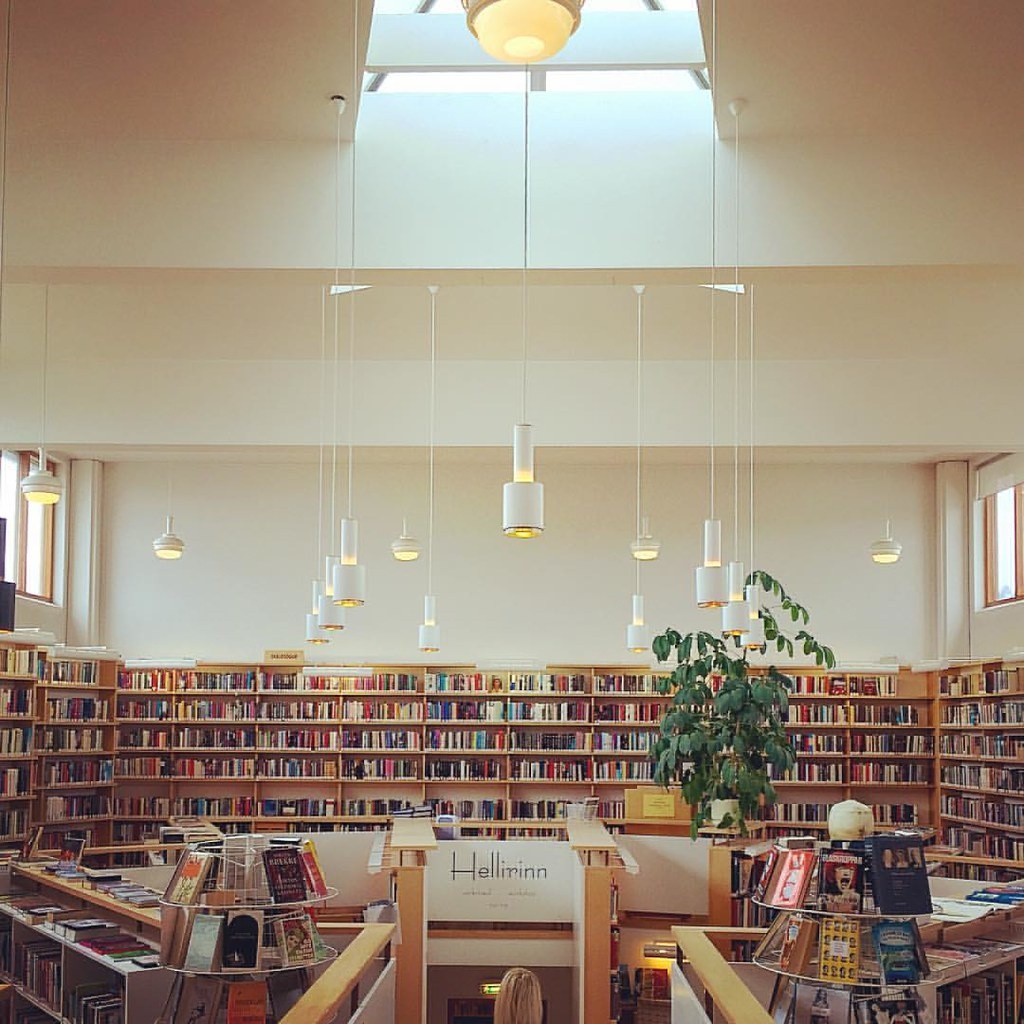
[307,1,365,644]
[502,66,544,539]
[153,478,184,560]
[461,0,585,66]
[696,0,765,649]
[626,285,660,653]
[392,284,439,654]
[870,514,902,565]
[20,282,63,506]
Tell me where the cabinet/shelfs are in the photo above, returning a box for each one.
[0,634,1024,1023]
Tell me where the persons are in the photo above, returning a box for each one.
[809,989,830,1024]
[817,851,860,913]
[493,968,542,1024]
[895,850,908,867]
[882,849,894,868]
[909,848,923,868]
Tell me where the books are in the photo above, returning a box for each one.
[0,646,1024,1024]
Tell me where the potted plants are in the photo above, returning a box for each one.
[652,570,837,841]
